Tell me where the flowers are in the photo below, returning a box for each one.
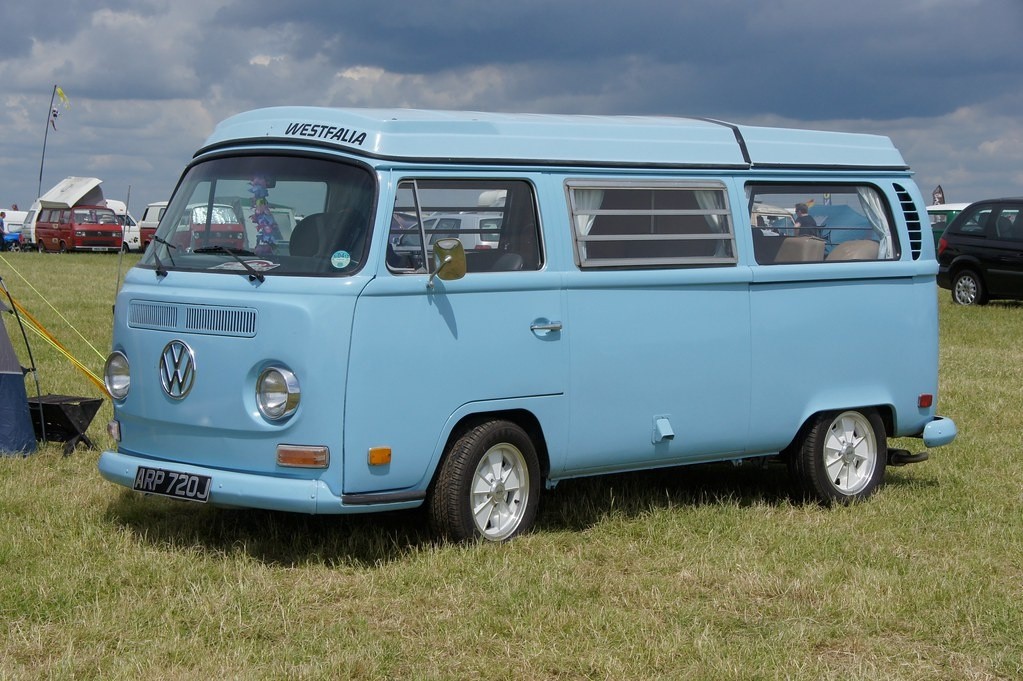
[247,176,279,256]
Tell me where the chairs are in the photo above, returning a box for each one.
[825,237,880,261]
[490,253,527,272]
[286,212,364,260]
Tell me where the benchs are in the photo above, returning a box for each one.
[754,234,825,263]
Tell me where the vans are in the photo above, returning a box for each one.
[96,107,958,553]
[138,201,243,253]
[750,202,798,238]
[35,206,124,255]
[0,177,142,256]
[923,197,1023,306]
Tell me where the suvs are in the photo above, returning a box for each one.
[392,213,503,258]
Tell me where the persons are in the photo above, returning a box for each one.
[0,212,6,252]
[793,203,817,237]
[11,205,19,210]
